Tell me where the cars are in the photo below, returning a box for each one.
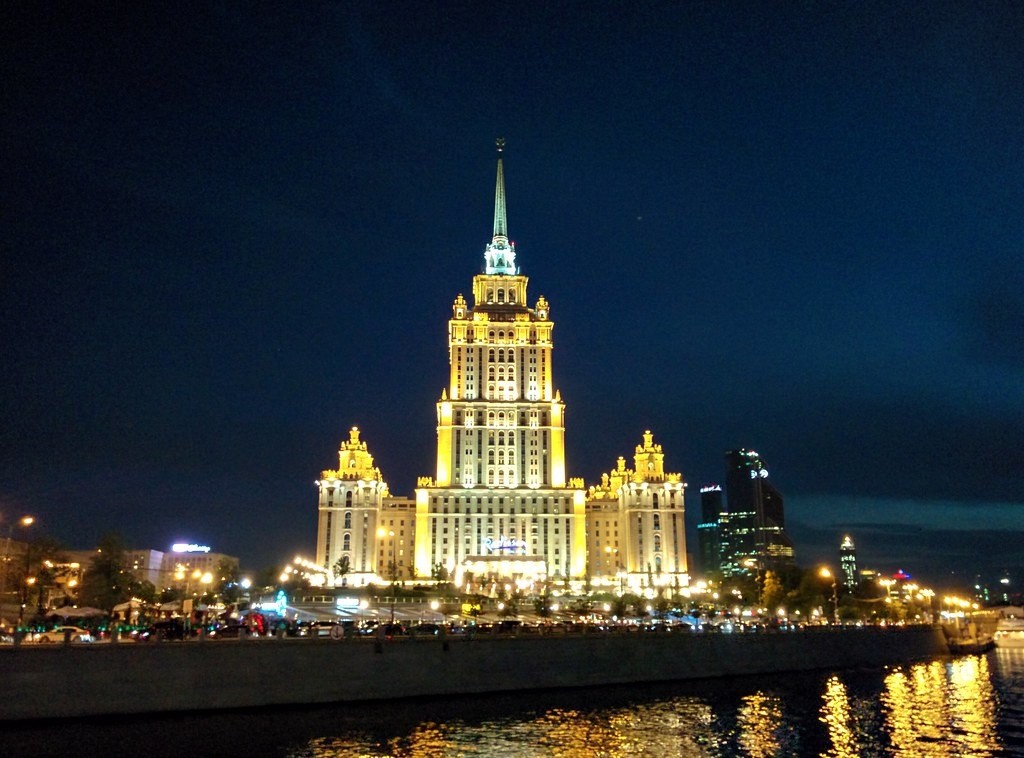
[128,621,200,643]
[359,622,443,637]
[209,623,257,640]
[33,626,91,644]
[297,620,338,637]
